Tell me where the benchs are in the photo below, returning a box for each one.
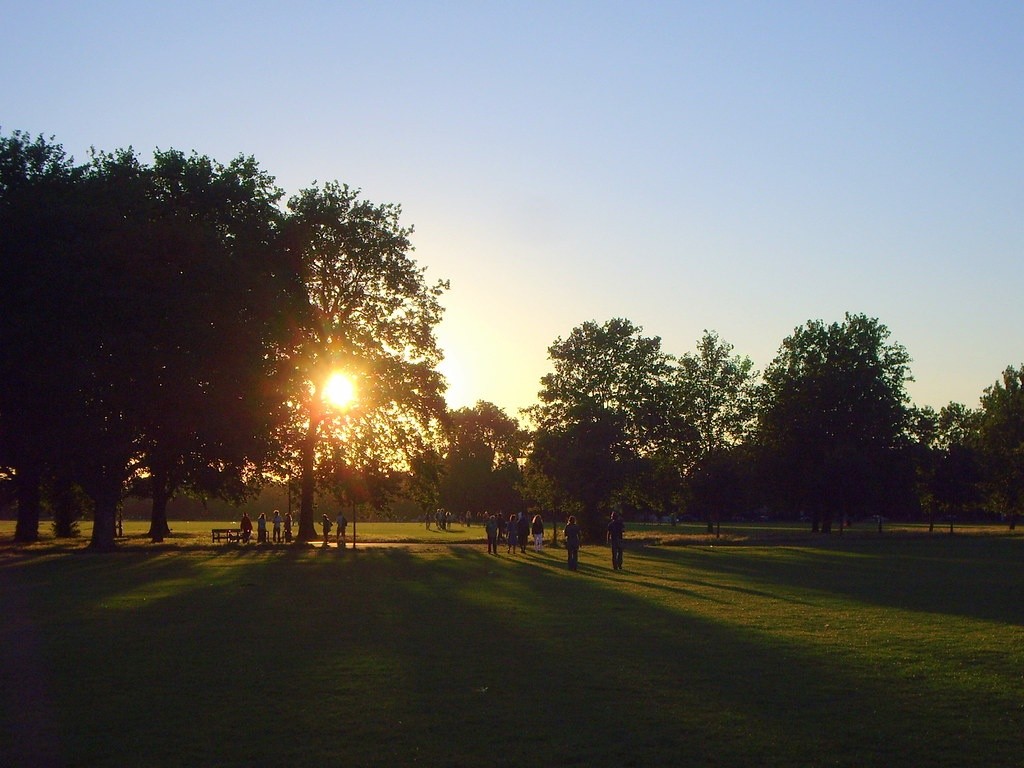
[211,527,243,543]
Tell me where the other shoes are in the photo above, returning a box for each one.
[618,565,622,570]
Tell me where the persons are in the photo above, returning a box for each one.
[283,513,291,543]
[606,511,625,571]
[318,512,332,547]
[425,508,543,555]
[257,513,266,543]
[564,516,581,571]
[336,511,347,547]
[272,510,284,543]
[240,512,253,543]
